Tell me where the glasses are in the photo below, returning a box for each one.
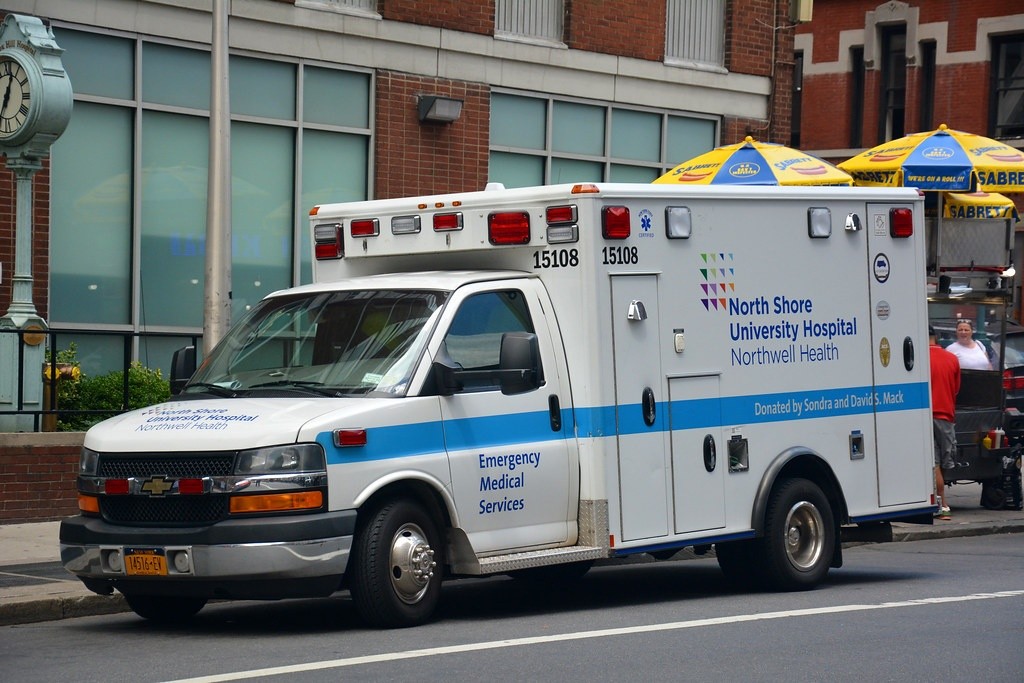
[958,319,971,323]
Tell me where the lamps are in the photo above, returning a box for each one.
[418,96,466,123]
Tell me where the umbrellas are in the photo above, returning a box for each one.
[836,124,1024,293]
[651,136,853,186]
[915,182,1021,224]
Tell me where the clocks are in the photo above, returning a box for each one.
[0,54,32,140]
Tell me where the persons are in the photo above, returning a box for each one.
[928,323,960,512]
[945,320,993,370]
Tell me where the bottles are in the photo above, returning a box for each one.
[982,426,1008,450]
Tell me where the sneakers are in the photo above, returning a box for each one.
[932,507,952,520]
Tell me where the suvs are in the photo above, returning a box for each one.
[930,320,1024,415]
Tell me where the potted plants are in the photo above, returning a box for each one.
[40,341,81,385]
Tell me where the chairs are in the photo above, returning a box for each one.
[313,341,356,366]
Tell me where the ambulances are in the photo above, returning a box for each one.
[59,180,940,632]
